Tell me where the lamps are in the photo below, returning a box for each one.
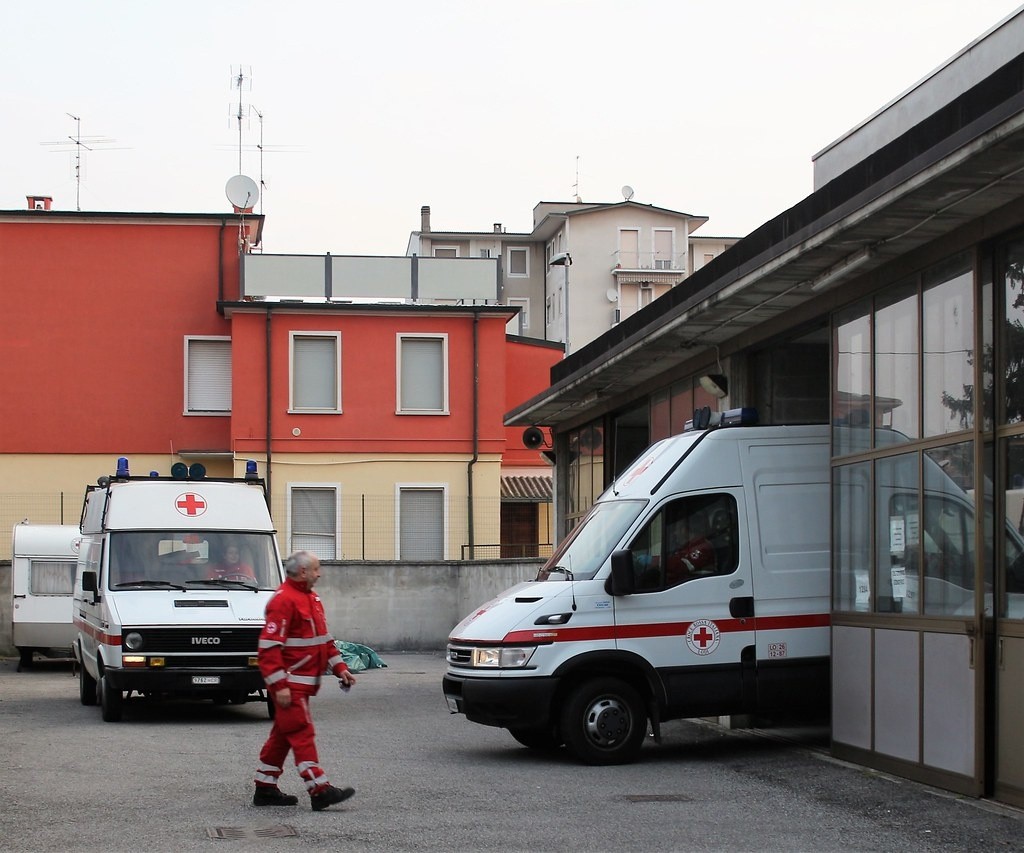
[699,372,728,400]
[579,391,602,407]
[539,449,555,466]
[810,245,878,291]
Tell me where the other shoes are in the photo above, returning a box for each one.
[254,785,299,806]
[310,785,356,812]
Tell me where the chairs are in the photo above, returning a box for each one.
[116,541,145,583]
[711,510,738,574]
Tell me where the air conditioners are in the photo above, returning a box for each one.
[640,282,653,290]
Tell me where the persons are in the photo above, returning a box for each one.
[206,544,256,581]
[637,509,718,588]
[254,551,355,810]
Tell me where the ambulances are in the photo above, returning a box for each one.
[434,406,832,766]
[70,454,290,723]
[832,424,1024,624]
